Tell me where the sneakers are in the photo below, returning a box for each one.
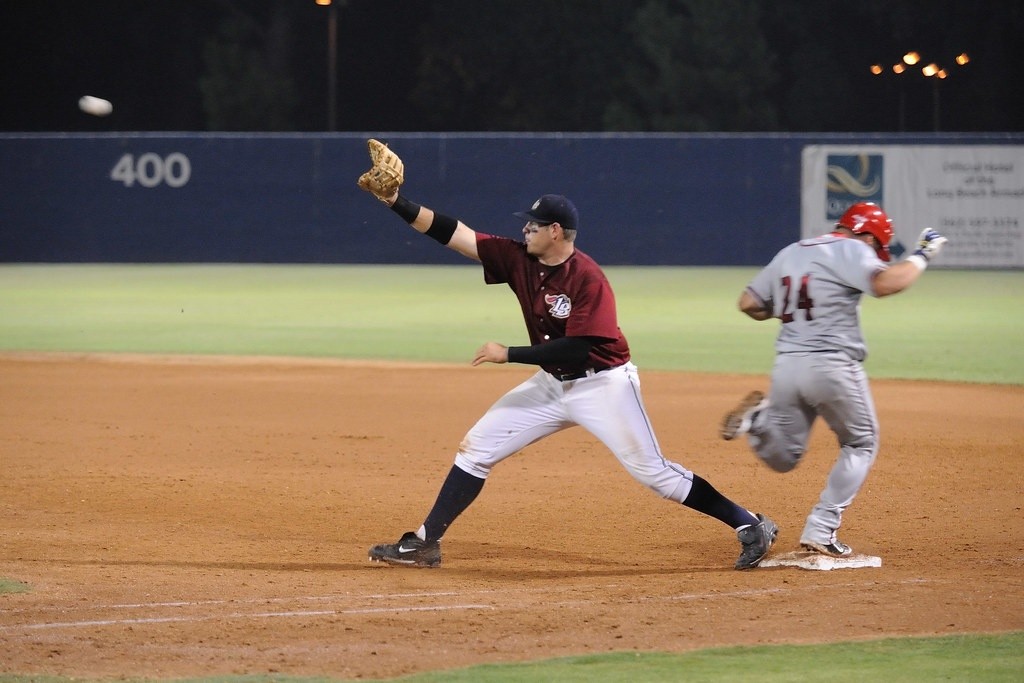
[367,530,443,568]
[720,390,769,440]
[800,537,855,557]
[735,512,779,569]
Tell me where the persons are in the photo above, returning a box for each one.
[723,203,948,558]
[356,140,777,570]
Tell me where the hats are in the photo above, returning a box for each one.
[512,195,580,232]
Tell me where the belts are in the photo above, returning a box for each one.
[552,367,608,382]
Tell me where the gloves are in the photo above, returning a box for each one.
[905,226,948,272]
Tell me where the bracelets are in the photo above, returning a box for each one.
[389,195,421,224]
[906,255,927,271]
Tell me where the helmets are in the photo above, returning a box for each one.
[835,202,896,262]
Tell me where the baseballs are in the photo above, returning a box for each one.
[78,95,113,118]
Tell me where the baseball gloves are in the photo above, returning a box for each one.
[356,139,405,203]
